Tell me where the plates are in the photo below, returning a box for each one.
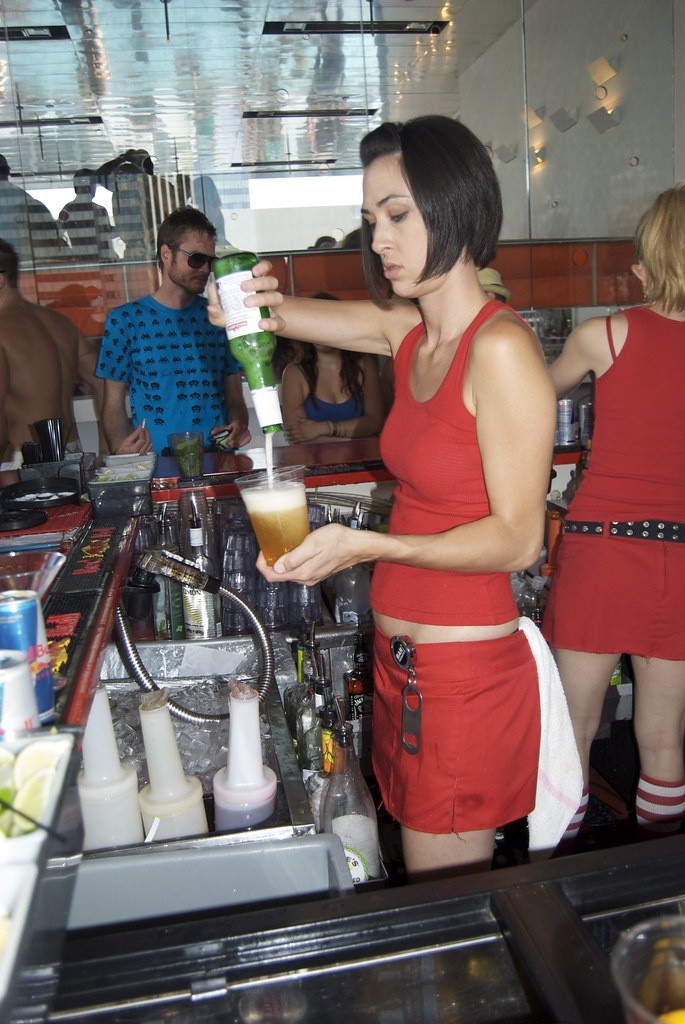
[88,451,156,485]
[0,732,75,999]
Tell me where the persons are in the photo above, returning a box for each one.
[94,205,253,458]
[10,201,74,261]
[95,148,180,261]
[540,186,684,845]
[0,237,111,471]
[59,168,126,261]
[0,154,74,258]
[476,268,512,304]
[206,116,560,877]
[40,283,105,337]
[282,290,384,444]
[176,175,225,236]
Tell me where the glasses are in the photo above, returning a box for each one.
[167,243,216,268]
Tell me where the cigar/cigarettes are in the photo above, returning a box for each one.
[141,419,146,430]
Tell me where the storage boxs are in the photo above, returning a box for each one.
[68,833,358,929]
[615,676,634,721]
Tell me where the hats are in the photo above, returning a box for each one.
[475,267,512,300]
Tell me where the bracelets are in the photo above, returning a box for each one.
[324,420,348,437]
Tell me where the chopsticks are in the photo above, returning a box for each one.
[21,418,65,465]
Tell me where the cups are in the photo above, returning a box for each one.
[169,431,205,480]
[136,491,325,632]
[233,465,312,567]
[609,913,685,1023]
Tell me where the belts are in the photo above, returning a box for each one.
[564,520,685,543]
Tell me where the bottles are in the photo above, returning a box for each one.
[281,640,381,885]
[324,502,389,623]
[508,439,593,631]
[152,521,185,642]
[76,683,278,851]
[211,251,285,434]
[181,518,223,640]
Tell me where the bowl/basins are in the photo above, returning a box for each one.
[0,551,66,599]
[0,477,79,509]
